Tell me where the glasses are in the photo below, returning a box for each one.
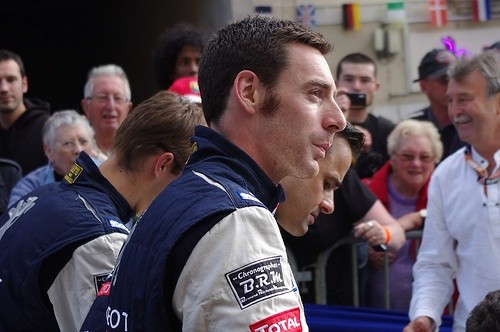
[86,92,131,105]
[392,152,434,162]
[425,76,449,86]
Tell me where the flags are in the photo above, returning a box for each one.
[343,3,361,31]
[429,0,448,28]
[255,6,271,17]
[296,5,316,27]
[472,0,492,22]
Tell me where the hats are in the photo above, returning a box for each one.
[168,77,202,103]
[413,48,458,83]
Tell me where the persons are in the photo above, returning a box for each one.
[82,64,132,160]
[131,23,204,108]
[80,14,347,332]
[274,121,365,237]
[0,90,208,332]
[334,52,396,180]
[410,49,466,158]
[361,119,460,316]
[403,51,500,332]
[8,109,106,207]
[0,50,52,178]
[279,168,405,304]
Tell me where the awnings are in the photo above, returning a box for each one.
[388,3,404,20]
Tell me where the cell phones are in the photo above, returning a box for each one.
[347,92,366,106]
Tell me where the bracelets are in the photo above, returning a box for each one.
[384,226,390,245]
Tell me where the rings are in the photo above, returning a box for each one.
[368,222,373,227]
